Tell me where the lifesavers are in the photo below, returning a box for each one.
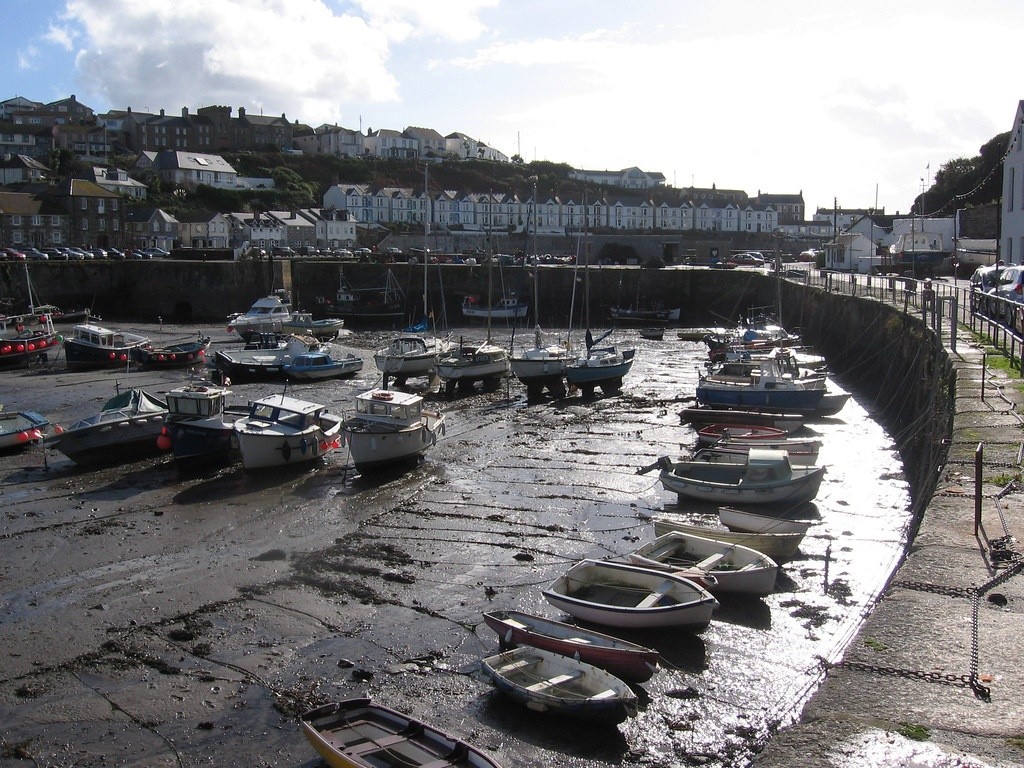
[372,392,394,401]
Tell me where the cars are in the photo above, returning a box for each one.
[798,250,816,262]
[728,254,764,267]
[301,246,372,258]
[745,252,765,261]
[970,263,1024,328]
[145,247,171,258]
[0,247,154,261]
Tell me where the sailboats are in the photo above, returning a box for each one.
[504,182,637,387]
[372,163,456,376]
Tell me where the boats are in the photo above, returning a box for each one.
[233,379,343,473]
[699,423,789,444]
[479,643,639,728]
[607,304,681,328]
[214,286,345,381]
[890,177,951,270]
[338,386,446,477]
[654,518,807,559]
[129,331,212,370]
[461,292,528,320]
[677,437,826,466]
[430,338,511,380]
[635,449,827,506]
[676,331,709,341]
[297,697,504,768]
[63,294,149,368]
[677,270,853,434]
[163,367,259,461]
[43,379,173,472]
[283,350,363,382]
[0,411,50,448]
[719,504,812,535]
[638,328,665,340]
[0,263,90,370]
[541,558,720,634]
[482,609,659,686]
[629,530,778,598]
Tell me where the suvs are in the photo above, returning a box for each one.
[272,247,296,258]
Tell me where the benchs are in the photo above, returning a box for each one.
[742,431,754,435]
[492,655,543,673]
[526,669,584,693]
[590,688,617,698]
[349,721,424,757]
[690,553,725,571]
[562,637,592,644]
[646,541,683,560]
[417,741,468,768]
[635,580,675,608]
